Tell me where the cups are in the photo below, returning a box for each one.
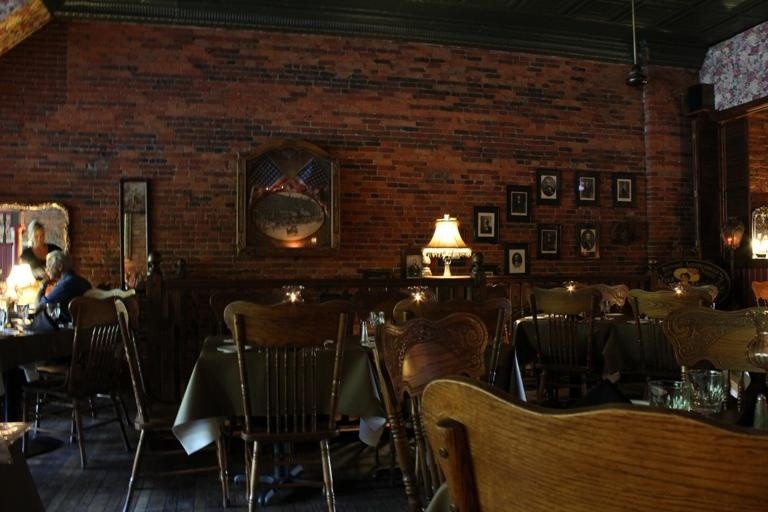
[46,303,60,323]
[17,305,29,319]
[645,365,731,413]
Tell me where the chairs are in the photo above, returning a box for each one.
[1,288,153,509]
[646,260,731,311]
[112,277,767,512]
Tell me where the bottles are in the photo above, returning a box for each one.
[361,321,368,344]
[379,311,385,324]
[370,312,378,328]
[352,311,360,335]
[752,394,768,430]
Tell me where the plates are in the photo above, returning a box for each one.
[627,319,650,325]
[215,344,252,354]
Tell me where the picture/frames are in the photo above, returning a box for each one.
[400,248,424,277]
[472,166,636,276]
[435,227,467,268]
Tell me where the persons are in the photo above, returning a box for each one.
[28,248,93,368]
[20,219,62,280]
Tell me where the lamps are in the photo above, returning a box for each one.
[718,216,748,303]
[5,264,38,309]
[423,213,473,279]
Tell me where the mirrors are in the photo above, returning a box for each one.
[0,198,70,286]
[116,174,152,292]
[748,202,766,265]
